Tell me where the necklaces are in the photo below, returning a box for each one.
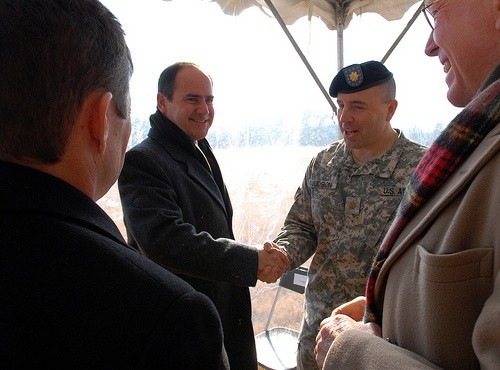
[352,148,366,163]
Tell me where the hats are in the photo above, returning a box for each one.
[328,60,393,97]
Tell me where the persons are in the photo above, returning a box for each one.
[118,62,291,369]
[257,61,435,369]
[314,0,500,370]
[0,1,232,370]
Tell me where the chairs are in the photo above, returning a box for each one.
[253,265,311,370]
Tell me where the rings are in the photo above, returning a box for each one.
[313,348,317,354]
